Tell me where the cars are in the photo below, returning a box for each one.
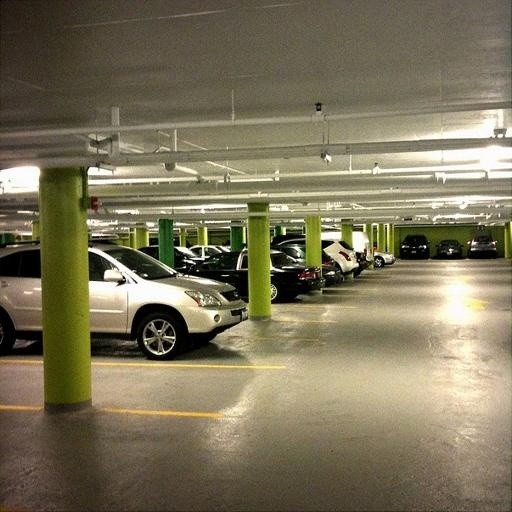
[184,247,325,306]
[275,237,371,284]
[1,237,249,360]
[138,245,202,272]
[373,251,396,269]
[188,242,232,258]
[435,240,464,258]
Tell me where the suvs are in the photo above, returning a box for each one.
[399,234,431,260]
[466,233,499,258]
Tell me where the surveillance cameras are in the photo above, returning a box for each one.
[320,151,332,164]
[164,162,175,172]
